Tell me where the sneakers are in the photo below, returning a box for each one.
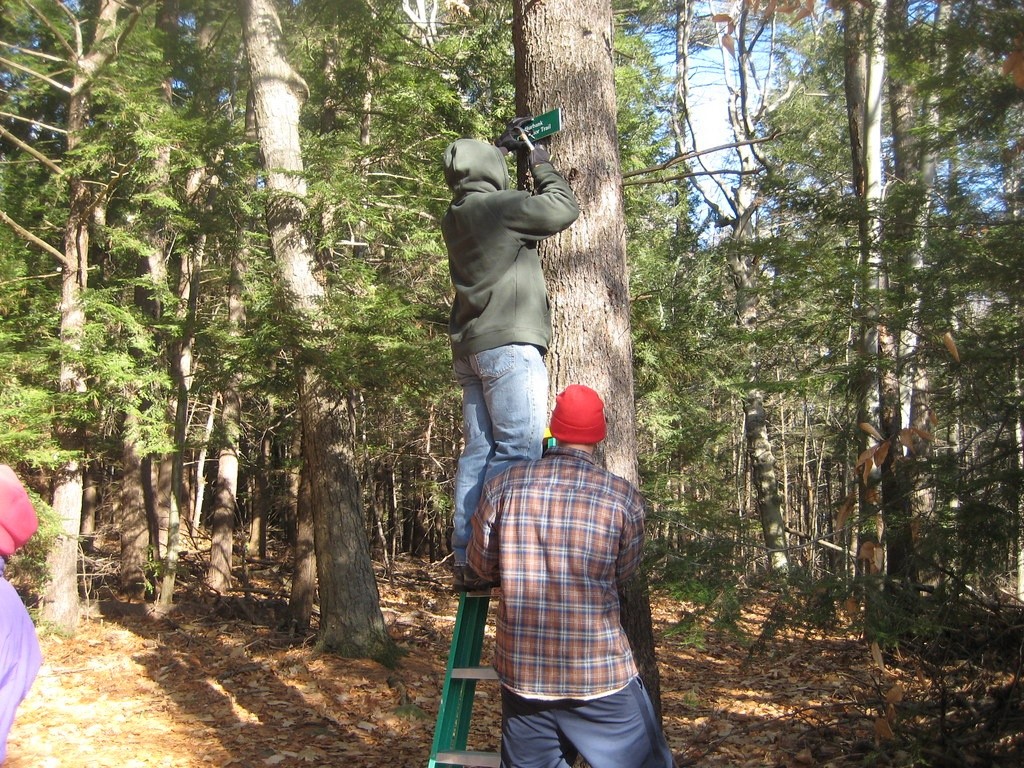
[461,565,500,589]
[451,567,470,592]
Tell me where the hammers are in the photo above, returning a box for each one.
[512,126,536,152]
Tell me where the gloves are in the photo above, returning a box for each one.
[529,144,551,169]
[494,115,533,153]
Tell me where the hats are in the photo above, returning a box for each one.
[550,383,606,443]
[0,464,40,555]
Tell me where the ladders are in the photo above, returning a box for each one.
[425,425,576,768]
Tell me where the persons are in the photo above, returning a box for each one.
[429,117,582,595]
[448,380,676,768]
[0,461,45,767]
[541,427,554,460]
